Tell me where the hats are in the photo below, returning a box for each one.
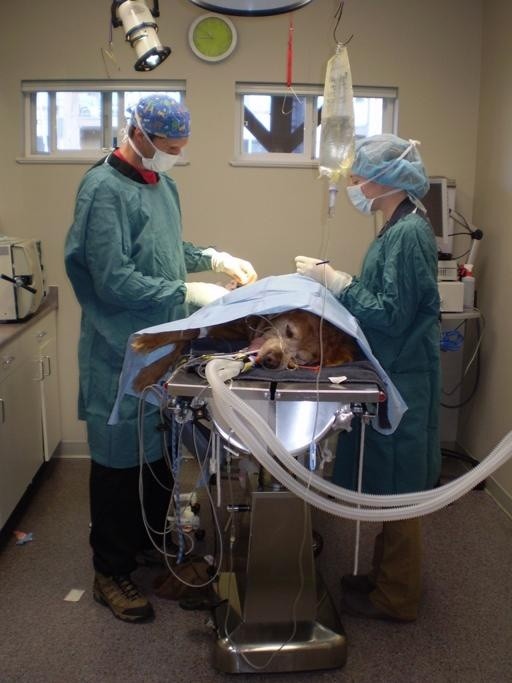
[351,133,430,198]
[127,96,191,138]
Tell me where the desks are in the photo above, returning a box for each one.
[160,363,387,675]
[438,306,482,458]
[323,468,326,471]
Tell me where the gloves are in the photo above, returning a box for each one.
[212,250,258,287]
[184,281,229,308]
[294,256,348,295]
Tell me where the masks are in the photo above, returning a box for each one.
[345,138,420,214]
[120,107,179,173]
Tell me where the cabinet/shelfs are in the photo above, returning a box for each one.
[0,285,63,518]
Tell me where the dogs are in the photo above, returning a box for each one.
[131,309,355,393]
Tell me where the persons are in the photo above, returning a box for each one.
[63,96,257,623]
[295,134,442,624]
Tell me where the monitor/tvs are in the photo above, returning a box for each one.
[416,176,457,260]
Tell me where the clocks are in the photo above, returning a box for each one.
[187,12,237,63]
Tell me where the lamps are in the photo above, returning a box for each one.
[109,0,172,73]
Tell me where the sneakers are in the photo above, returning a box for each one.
[93,572,153,622]
[135,541,179,565]
[341,592,414,622]
[341,574,369,593]
